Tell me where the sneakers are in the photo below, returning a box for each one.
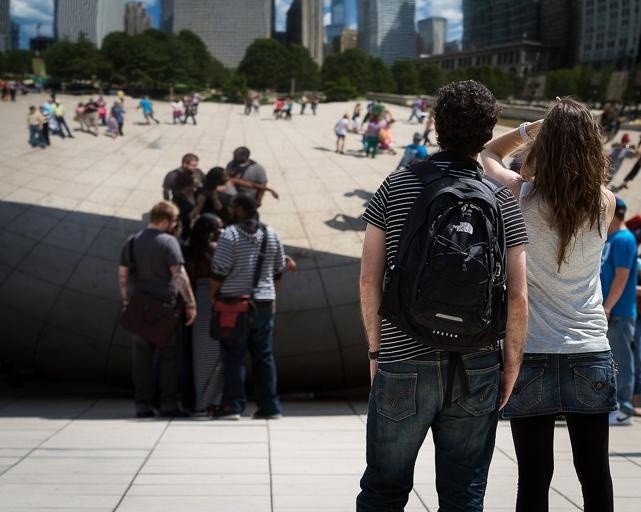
[610,410,634,425]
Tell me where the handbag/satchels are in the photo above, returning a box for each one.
[120,294,179,348]
[210,293,255,340]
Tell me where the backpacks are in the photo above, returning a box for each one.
[377,158,508,352]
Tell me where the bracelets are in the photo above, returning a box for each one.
[367,350,379,361]
[520,121,534,143]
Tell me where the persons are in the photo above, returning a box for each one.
[604,133,638,188]
[171,98,185,124]
[332,94,436,158]
[624,216,641,418]
[621,154,641,195]
[349,79,531,510]
[240,90,319,121]
[135,95,161,125]
[1,78,29,103]
[597,102,621,146]
[184,96,197,126]
[605,196,640,426]
[28,88,127,147]
[398,132,429,171]
[115,146,299,421]
[477,97,622,510]
